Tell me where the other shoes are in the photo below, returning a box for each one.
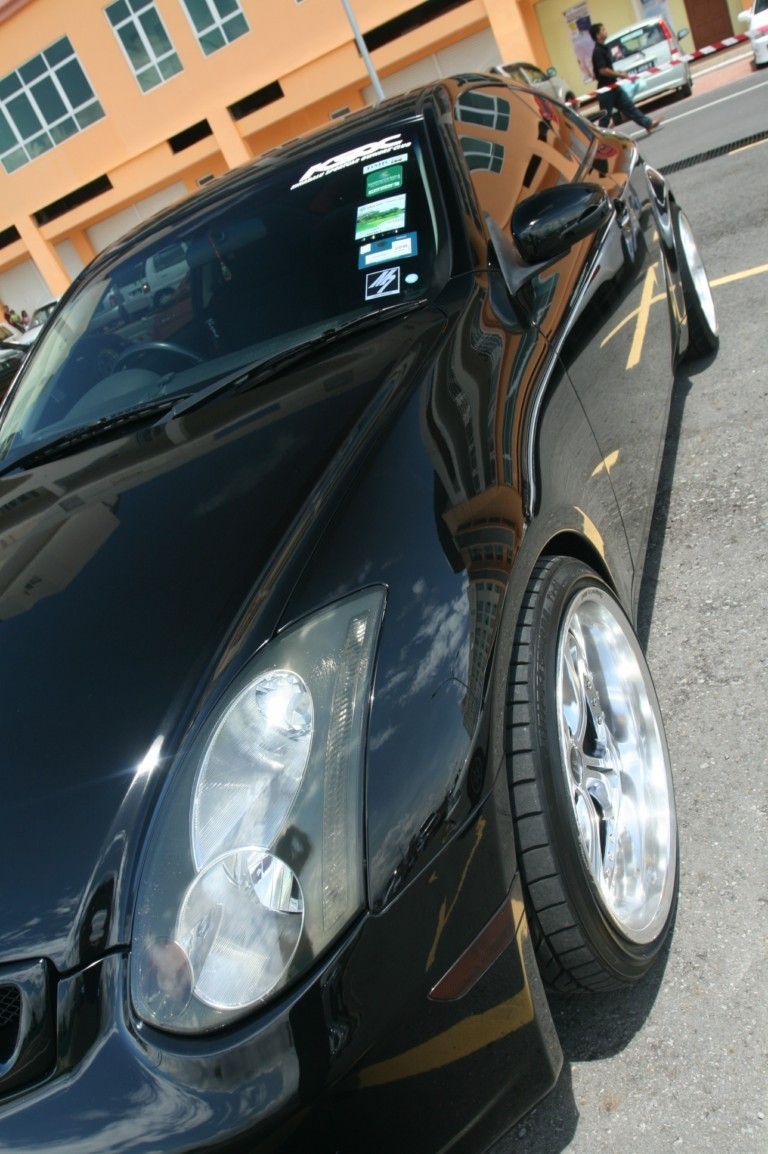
[646,117,660,131]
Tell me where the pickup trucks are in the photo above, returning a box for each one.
[490,64,578,110]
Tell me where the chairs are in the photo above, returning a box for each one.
[198,203,353,358]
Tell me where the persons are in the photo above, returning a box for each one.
[587,22,661,134]
[3,304,30,333]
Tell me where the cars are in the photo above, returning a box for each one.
[738,1,768,65]
[0,64,720,1154]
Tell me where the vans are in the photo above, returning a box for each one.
[598,15,693,125]
